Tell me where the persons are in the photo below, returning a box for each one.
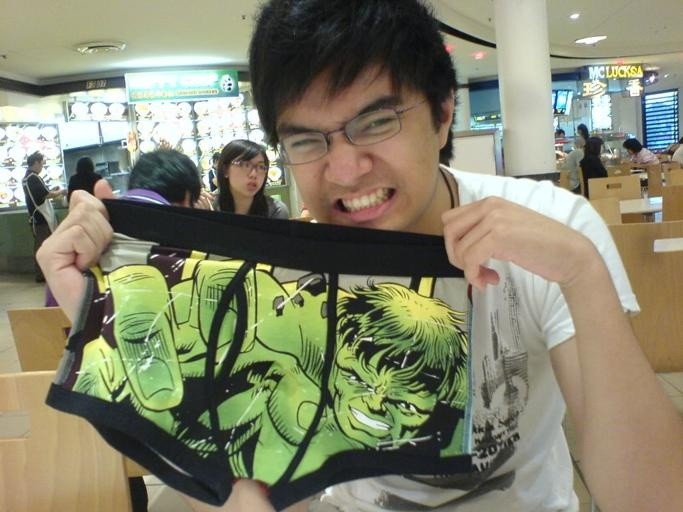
[192,139,290,221]
[22,152,69,283]
[665,136,683,164]
[34,1,682,512]
[40,148,201,309]
[67,156,102,202]
[553,123,589,193]
[208,152,221,192]
[579,136,608,198]
[622,138,660,173]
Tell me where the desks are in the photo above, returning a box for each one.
[0,207,69,276]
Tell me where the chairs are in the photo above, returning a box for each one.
[0,371,132,512]
[7,307,157,511]
[556,151,683,373]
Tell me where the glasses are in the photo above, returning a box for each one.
[270,98,427,165]
[232,159,268,173]
